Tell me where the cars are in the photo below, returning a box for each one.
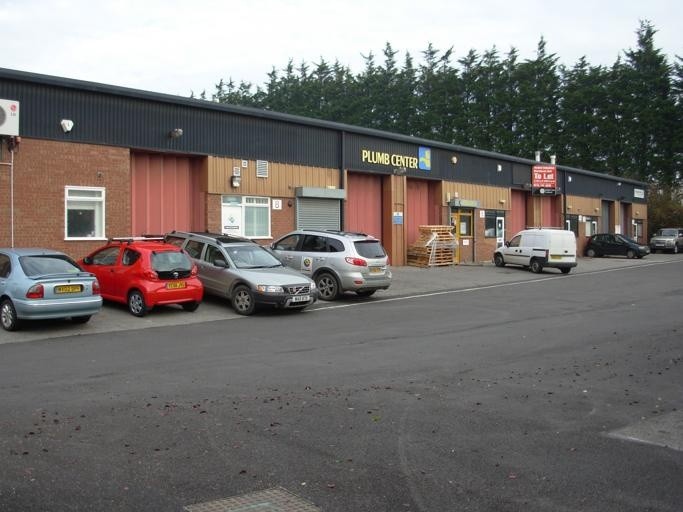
[75,235,204,317]
[0,247,104,332]
[650,228,683,254]
[584,234,650,259]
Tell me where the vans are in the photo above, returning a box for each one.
[492,229,577,273]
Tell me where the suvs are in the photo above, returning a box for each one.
[259,229,392,302]
[162,230,318,316]
